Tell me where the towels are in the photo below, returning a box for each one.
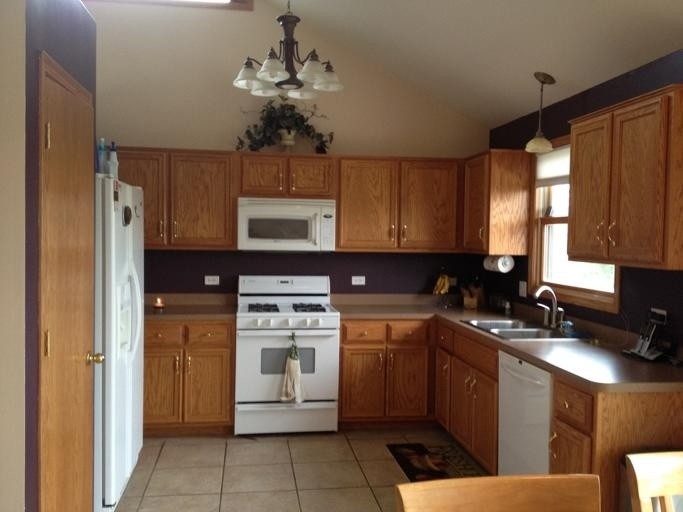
[277,355,304,408]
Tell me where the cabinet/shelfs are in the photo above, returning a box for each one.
[463,146,530,258]
[338,317,435,430]
[143,318,234,439]
[107,146,236,251]
[564,80,682,273]
[241,149,338,200]
[548,377,683,512]
[339,153,464,255]
[433,321,451,434]
[451,329,498,476]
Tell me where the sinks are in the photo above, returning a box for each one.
[468,319,534,329]
[489,327,579,341]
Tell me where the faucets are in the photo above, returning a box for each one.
[532,284,559,330]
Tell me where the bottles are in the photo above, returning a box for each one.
[504,302,510,314]
[96,137,106,175]
[108,141,118,179]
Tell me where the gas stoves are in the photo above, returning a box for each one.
[235,291,339,328]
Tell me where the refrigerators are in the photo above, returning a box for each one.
[93,174,144,512]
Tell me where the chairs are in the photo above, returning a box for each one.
[624,451,681,512]
[393,472,603,512]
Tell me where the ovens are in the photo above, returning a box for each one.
[233,330,340,436]
[496,348,551,476]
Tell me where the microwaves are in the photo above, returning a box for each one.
[236,196,335,253]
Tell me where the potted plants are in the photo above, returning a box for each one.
[234,93,334,147]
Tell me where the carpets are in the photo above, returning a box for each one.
[386,438,491,483]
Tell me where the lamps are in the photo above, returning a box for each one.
[525,65,562,155]
[227,0,344,99]
